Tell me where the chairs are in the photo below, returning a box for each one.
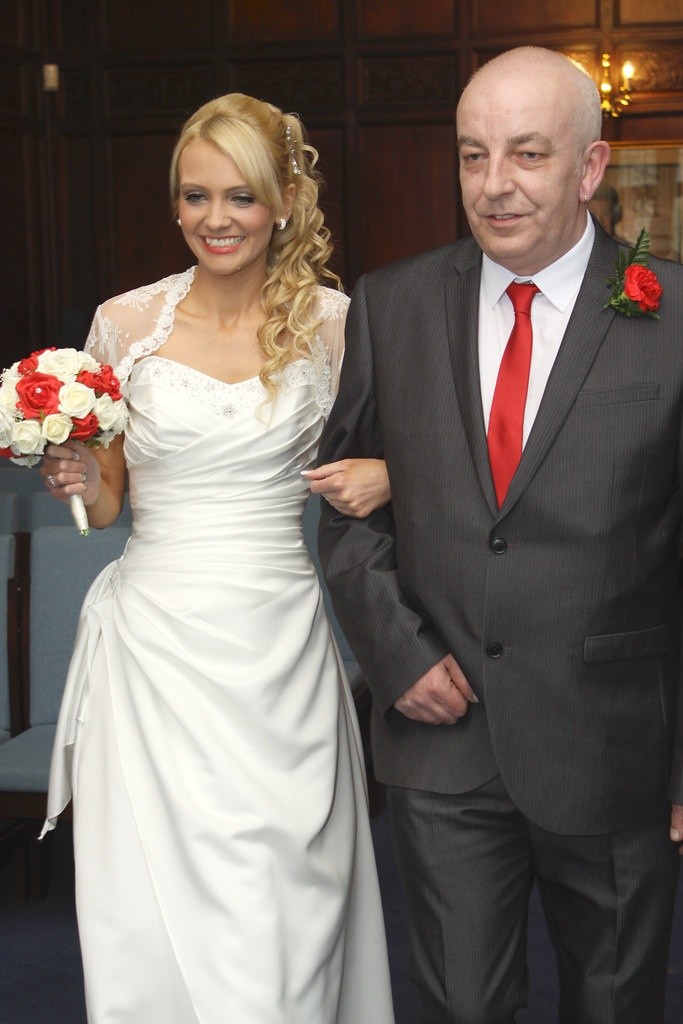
[0,460,132,898]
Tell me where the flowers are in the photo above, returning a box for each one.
[602,226,664,320]
[0,346,129,537]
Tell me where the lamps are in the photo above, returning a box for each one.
[599,52,635,119]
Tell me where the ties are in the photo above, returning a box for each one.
[487,283,541,511]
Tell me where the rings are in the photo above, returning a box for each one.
[47,475,56,487]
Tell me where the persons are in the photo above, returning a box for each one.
[304,47,683,1024]
[587,185,632,246]
[41,94,395,1024]
